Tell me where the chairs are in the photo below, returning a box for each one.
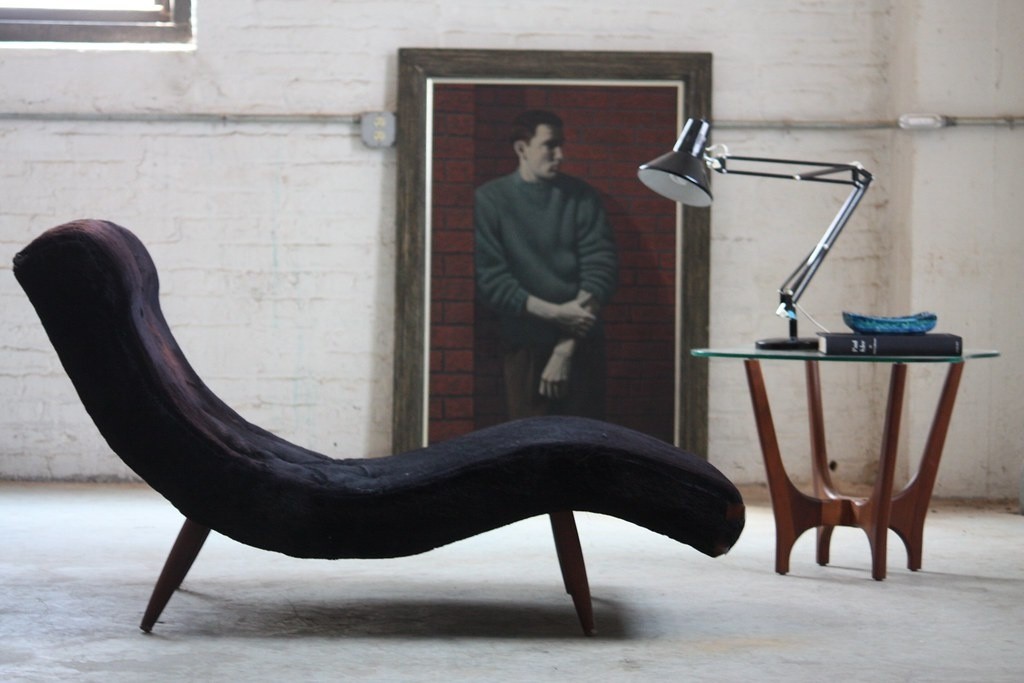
[12,216,748,640]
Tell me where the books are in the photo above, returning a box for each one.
[817,332,963,357]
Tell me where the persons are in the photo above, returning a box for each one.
[473,109,620,421]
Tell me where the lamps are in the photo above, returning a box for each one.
[635,117,875,355]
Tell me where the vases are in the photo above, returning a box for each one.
[387,44,715,461]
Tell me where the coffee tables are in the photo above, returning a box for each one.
[689,343,1002,582]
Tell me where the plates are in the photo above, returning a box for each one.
[843,312,936,332]
